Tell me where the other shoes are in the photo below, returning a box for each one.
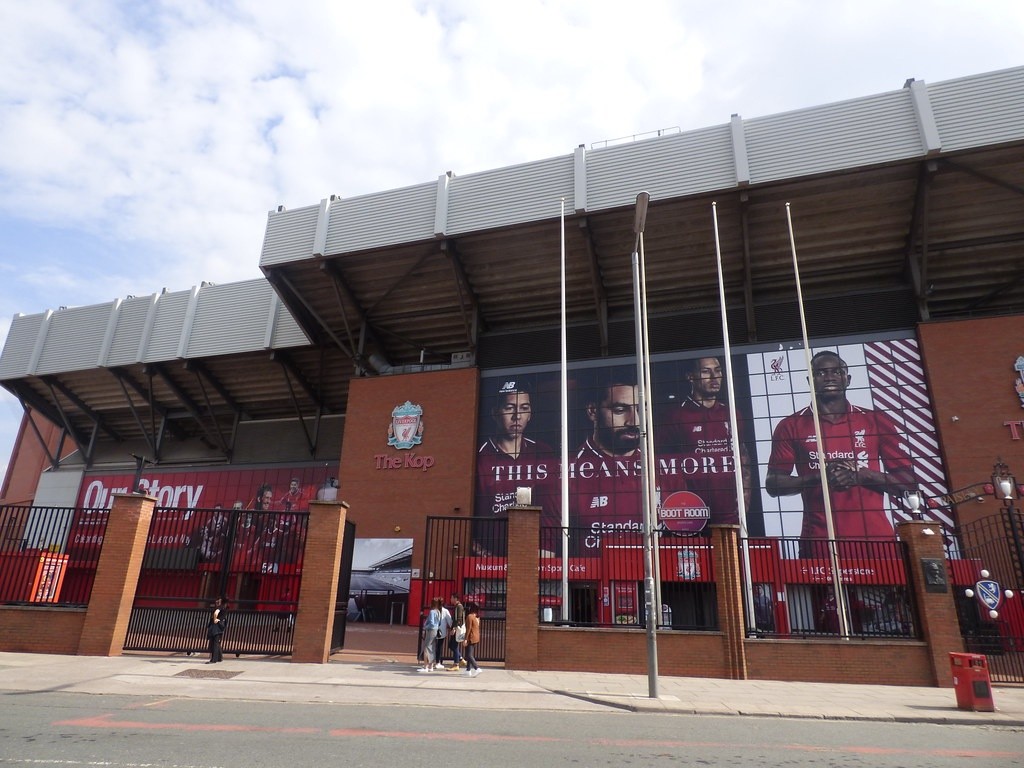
[435,663,445,668]
[217,659,223,662]
[206,661,216,664]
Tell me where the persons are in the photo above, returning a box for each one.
[200,478,307,563]
[472,351,919,558]
[417,592,483,678]
[205,596,229,664]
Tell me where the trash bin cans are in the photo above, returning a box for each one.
[948,652,996,712]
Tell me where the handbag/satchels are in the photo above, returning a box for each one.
[455,624,466,642]
[437,629,442,638]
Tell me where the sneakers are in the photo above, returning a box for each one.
[417,667,429,672]
[428,667,434,671]
[461,671,471,677]
[471,668,483,678]
[459,658,467,667]
[446,664,459,671]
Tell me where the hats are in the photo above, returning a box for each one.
[497,377,532,395]
